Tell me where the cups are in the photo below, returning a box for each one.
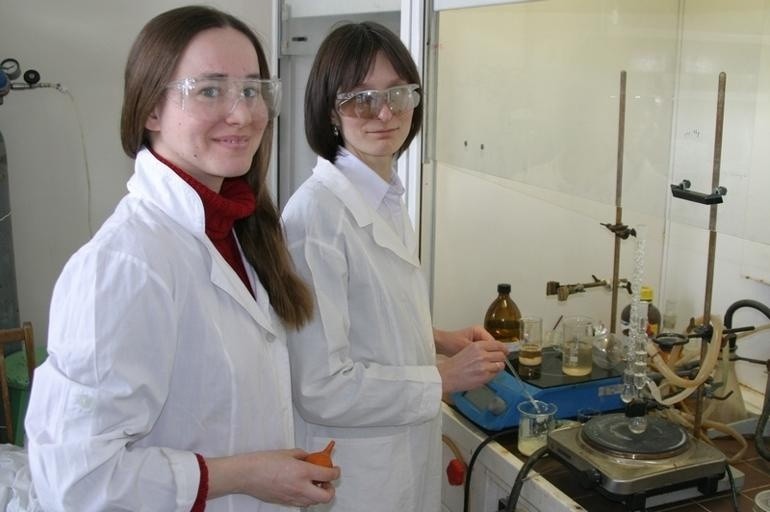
[519,316,544,379]
[562,314,595,378]
[516,399,558,457]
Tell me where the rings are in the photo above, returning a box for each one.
[495,362,503,373]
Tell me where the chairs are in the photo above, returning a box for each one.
[0,321,40,465]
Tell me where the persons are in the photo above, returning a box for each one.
[23,6,341,512]
[279,21,511,512]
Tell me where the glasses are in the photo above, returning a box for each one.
[334,83,420,119]
[166,78,282,122]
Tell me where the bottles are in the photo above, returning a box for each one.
[620,286,661,347]
[485,283,523,342]
